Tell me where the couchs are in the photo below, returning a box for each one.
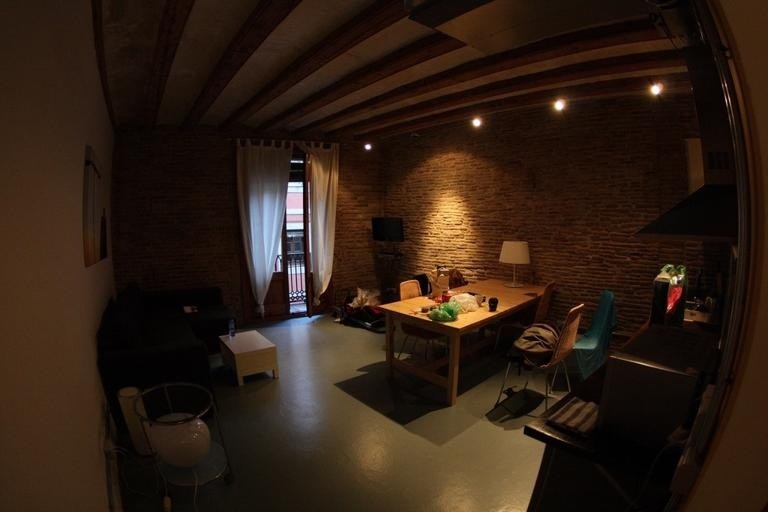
[97,286,237,397]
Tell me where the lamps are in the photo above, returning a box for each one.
[499,238,531,289]
[116,387,157,457]
[133,383,234,499]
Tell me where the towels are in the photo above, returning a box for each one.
[545,394,600,437]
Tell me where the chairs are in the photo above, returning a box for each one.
[397,279,426,361]
[568,288,615,385]
[492,277,556,357]
[495,304,584,411]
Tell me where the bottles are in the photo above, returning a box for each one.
[229,320,236,339]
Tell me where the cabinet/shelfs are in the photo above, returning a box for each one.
[524,310,721,512]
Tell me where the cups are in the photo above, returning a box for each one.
[489,298,498,312]
[442,290,455,303]
